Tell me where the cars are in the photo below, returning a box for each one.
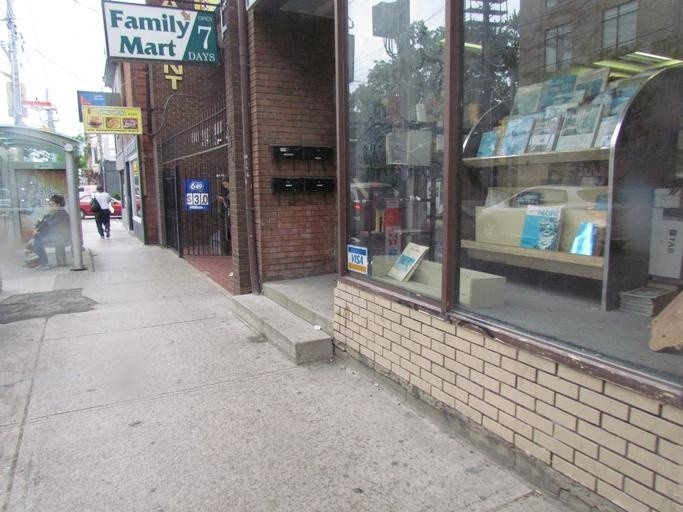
[0,188,14,221]
[79,193,121,218]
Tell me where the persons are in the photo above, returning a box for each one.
[90,185,112,240]
[31,194,71,271]
[214,176,230,239]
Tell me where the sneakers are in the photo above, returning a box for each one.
[227,271,234,277]
[35,264,51,270]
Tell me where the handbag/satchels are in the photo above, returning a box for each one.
[89,193,101,213]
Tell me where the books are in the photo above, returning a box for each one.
[385,240,431,283]
[474,66,641,257]
[616,286,674,318]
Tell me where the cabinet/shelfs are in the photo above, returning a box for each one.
[458,65,683,312]
[352,120,438,263]
